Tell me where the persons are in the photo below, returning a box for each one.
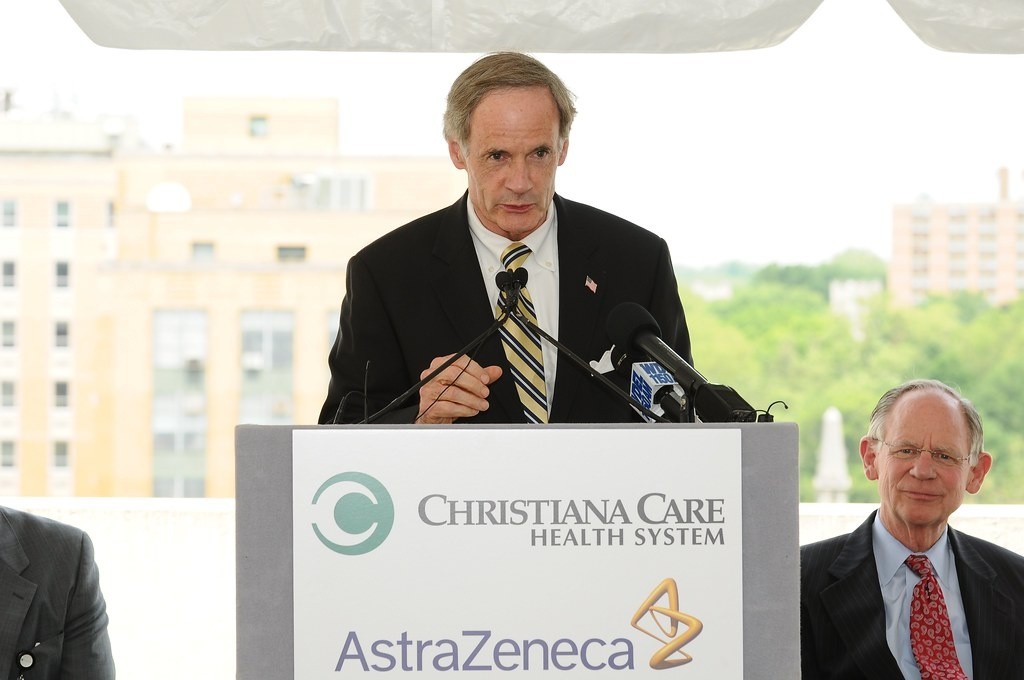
[799,378,1024,679]
[317,50,694,424]
[0,505,117,680]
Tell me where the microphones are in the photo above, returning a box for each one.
[354,265,757,425]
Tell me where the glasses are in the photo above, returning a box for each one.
[873,439,970,465]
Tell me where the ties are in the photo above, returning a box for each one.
[904,555,968,680]
[496,242,548,424]
